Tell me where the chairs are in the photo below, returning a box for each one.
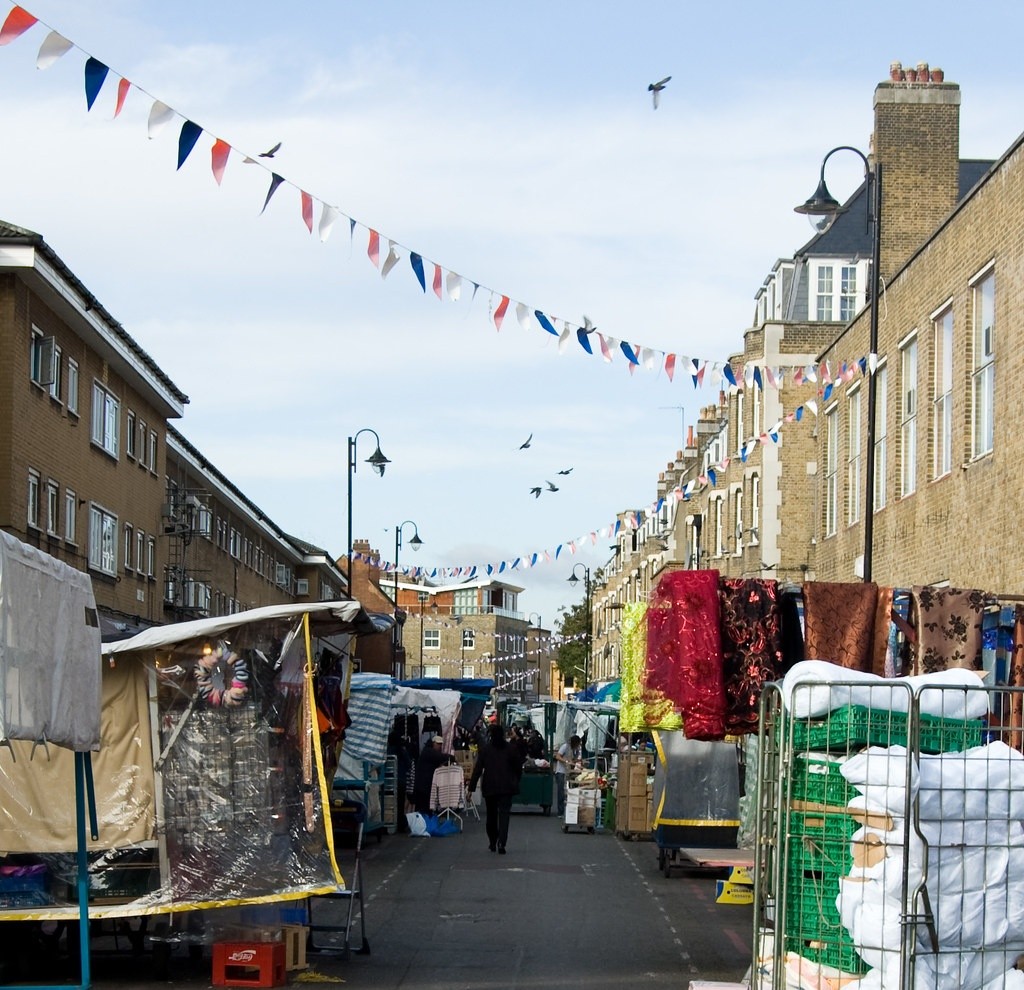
[429,765,465,810]
[307,797,371,959]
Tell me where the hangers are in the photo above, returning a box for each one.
[425,711,439,718]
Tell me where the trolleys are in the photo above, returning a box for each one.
[561,769,599,833]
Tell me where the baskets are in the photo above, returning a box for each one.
[771,702,984,753]
[772,808,872,975]
[0,871,47,909]
[72,881,148,898]
[240,906,306,926]
[785,756,862,809]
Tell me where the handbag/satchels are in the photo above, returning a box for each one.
[406,812,431,837]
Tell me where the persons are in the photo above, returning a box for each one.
[411,736,456,818]
[471,723,652,855]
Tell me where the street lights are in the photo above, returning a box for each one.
[566,562,590,702]
[527,611,541,703]
[420,594,439,679]
[348,428,392,600]
[393,521,425,677]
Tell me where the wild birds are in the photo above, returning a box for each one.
[258,142,284,161]
[382,527,390,534]
[519,434,536,452]
[530,466,576,501]
[648,76,672,111]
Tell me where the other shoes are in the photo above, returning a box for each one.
[498,844,505,854]
[558,816,565,819]
[488,844,496,852]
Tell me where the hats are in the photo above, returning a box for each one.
[433,736,445,744]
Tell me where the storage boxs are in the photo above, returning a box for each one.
[616,752,654,832]
[212,941,286,988]
[578,808,597,827]
[776,703,983,973]
